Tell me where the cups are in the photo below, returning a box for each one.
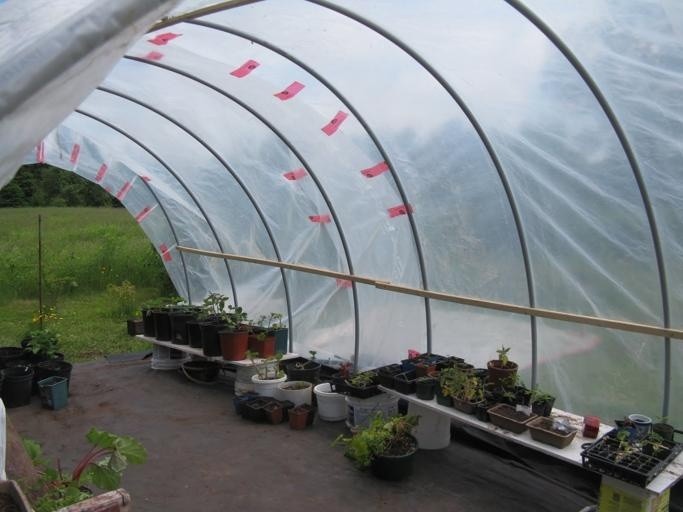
[628,414,653,434]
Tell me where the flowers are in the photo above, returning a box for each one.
[20,303,65,360]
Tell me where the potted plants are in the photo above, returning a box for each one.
[378,345,580,449]
[229,345,400,436]
[582,413,682,489]
[13,426,148,511]
[125,289,290,361]
[327,408,423,484]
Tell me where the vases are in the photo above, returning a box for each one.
[0,336,73,413]
